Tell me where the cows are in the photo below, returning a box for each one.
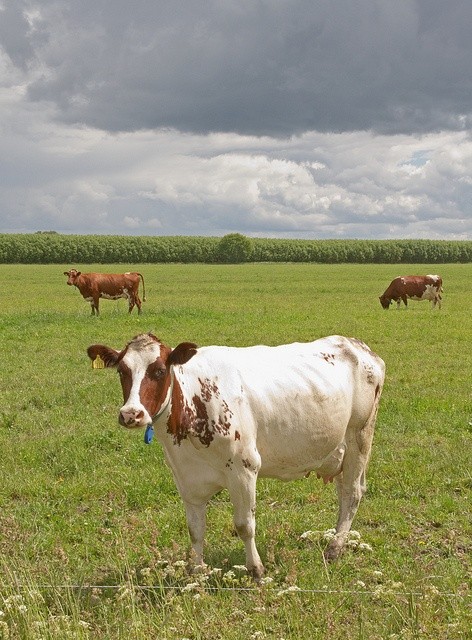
[379,274,445,310]
[85,333,387,579]
[63,269,147,316]
